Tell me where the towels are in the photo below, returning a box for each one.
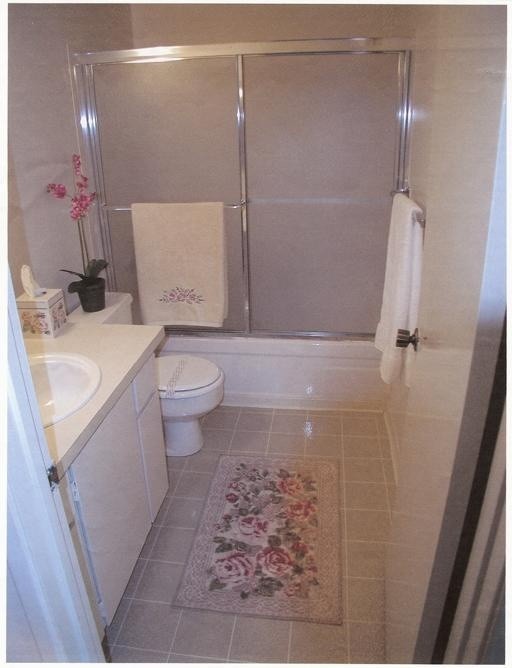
[375,193,425,386]
[130,201,230,328]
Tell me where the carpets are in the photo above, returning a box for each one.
[168,453,343,625]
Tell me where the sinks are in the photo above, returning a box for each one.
[27,351,102,430]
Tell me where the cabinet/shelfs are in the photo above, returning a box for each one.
[57,350,171,639]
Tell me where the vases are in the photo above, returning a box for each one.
[78,277,106,311]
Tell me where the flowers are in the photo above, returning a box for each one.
[45,149,108,292]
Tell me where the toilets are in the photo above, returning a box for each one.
[64,291,226,458]
